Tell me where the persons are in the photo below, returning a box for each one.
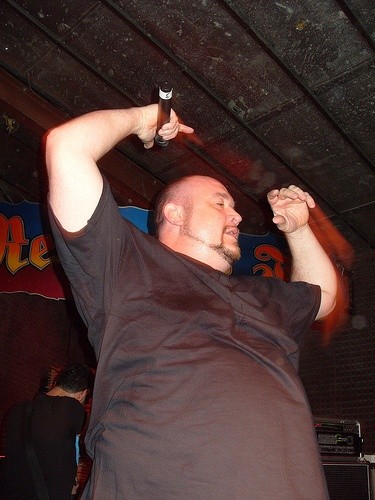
[1,363,94,500]
[45,104,339,500]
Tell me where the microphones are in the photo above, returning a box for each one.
[155,81,173,147]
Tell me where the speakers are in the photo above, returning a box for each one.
[320,461,372,499]
